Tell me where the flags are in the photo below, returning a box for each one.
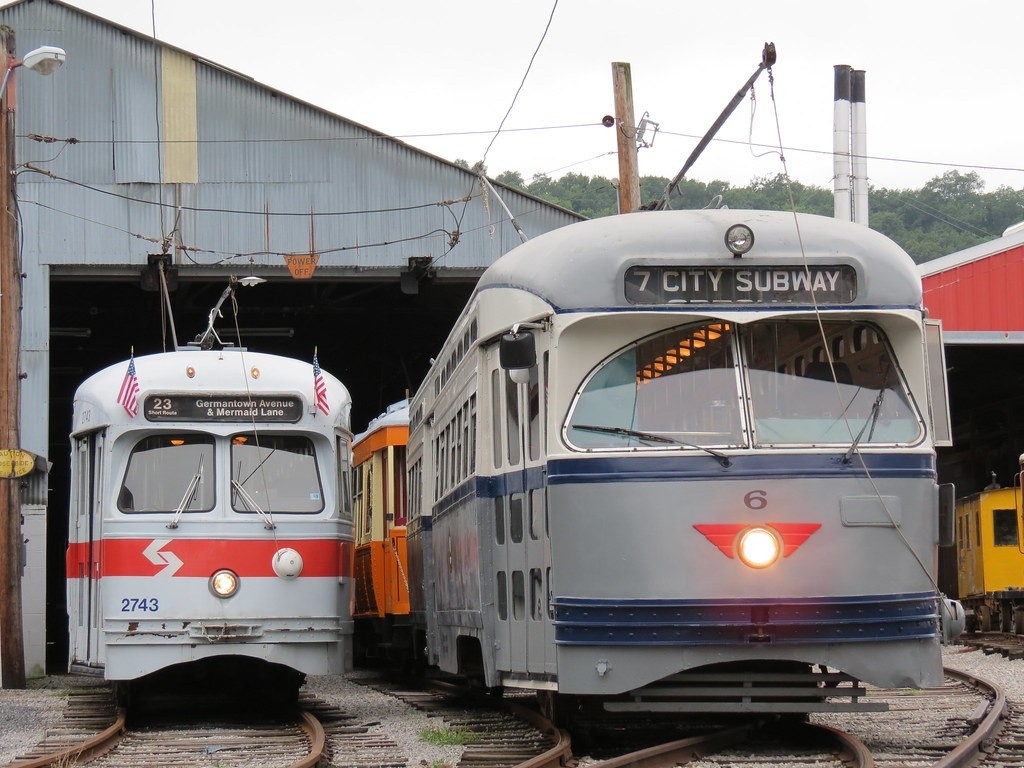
[116,355,141,418]
[313,355,330,416]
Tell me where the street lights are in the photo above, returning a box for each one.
[1,47,66,689]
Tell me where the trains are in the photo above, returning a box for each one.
[354,205,964,740]
[65,337,356,715]
[937,454,1024,635]
